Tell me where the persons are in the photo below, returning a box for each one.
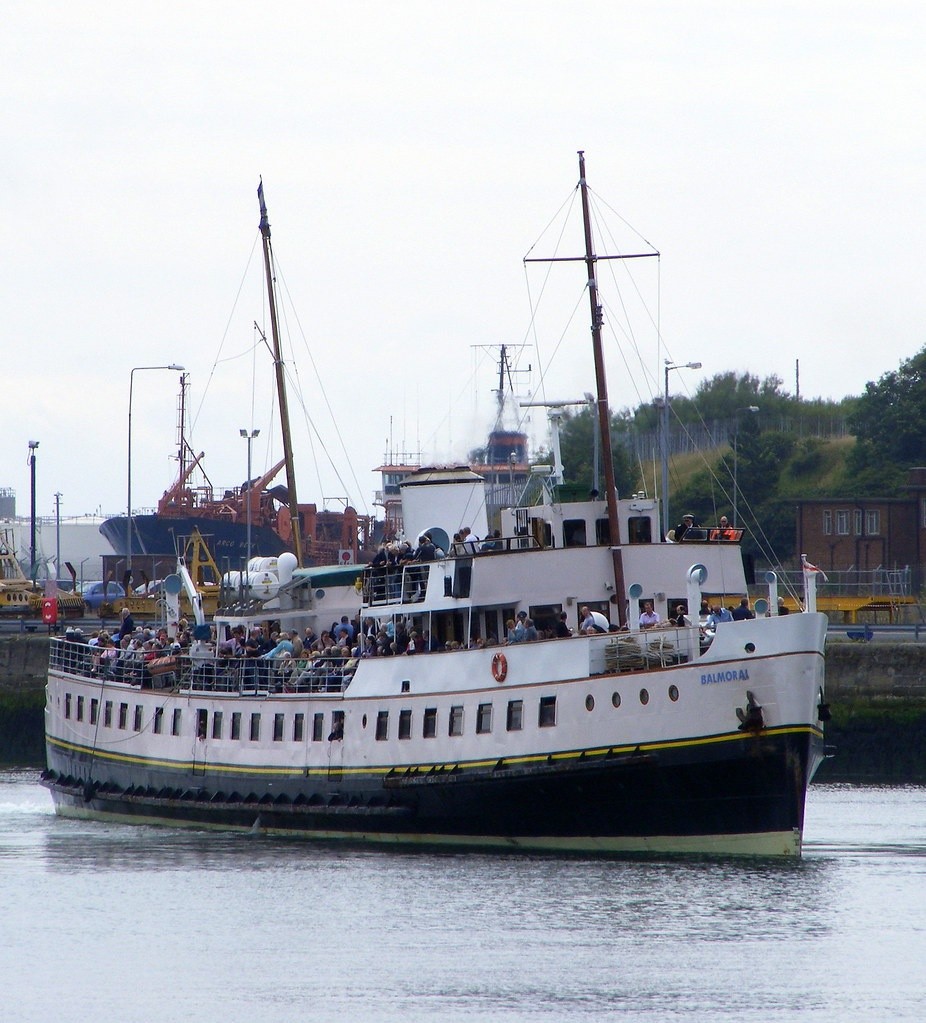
[371,527,503,606]
[674,513,733,542]
[75,608,437,693]
[445,595,790,652]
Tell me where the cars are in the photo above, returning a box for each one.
[36,578,77,592]
[132,580,185,598]
[182,580,222,588]
[69,581,126,615]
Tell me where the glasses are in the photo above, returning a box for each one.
[722,521,726,522]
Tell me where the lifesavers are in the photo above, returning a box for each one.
[83,780,93,803]
[492,653,507,682]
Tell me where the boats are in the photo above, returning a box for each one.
[45,148,829,863]
[372,347,561,548]
[0,524,86,621]
[99,371,400,584]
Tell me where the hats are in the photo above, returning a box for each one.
[92,631,99,638]
[682,513,695,520]
[75,628,83,634]
[408,631,418,638]
[66,626,75,634]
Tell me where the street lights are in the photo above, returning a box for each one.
[127,364,186,584]
[29,440,40,581]
[661,362,702,543]
[239,428,260,562]
[733,406,760,529]
